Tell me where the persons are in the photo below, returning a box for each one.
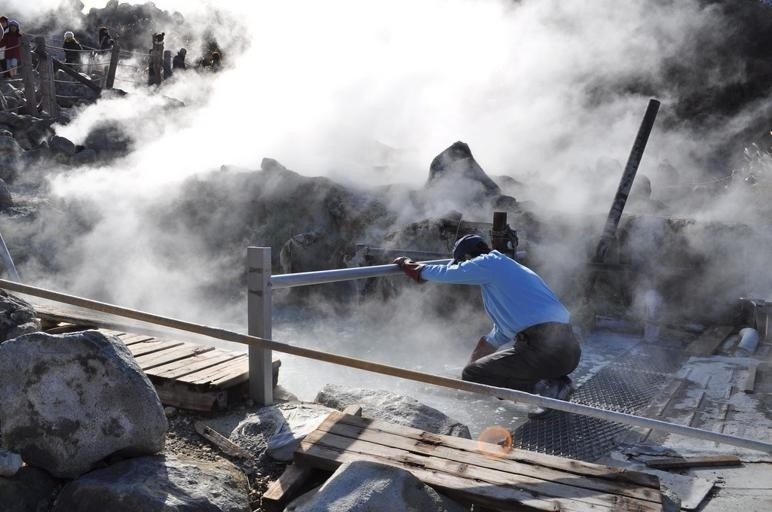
[1,15,22,77]
[63,31,83,70]
[173,48,186,70]
[392,234,582,419]
[101,33,116,49]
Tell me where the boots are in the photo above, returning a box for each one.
[528,376,574,418]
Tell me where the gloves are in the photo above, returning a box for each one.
[466,336,498,365]
[392,256,428,282]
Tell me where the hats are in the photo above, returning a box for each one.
[446,234,488,266]
